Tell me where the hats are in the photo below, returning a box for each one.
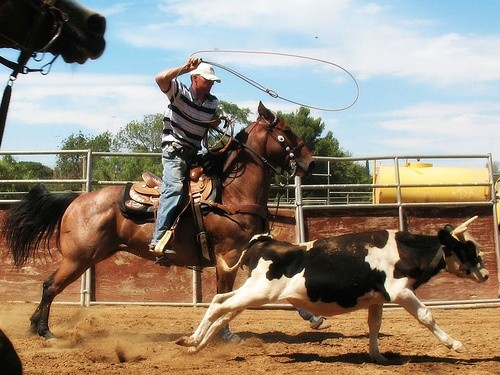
[189,63,221,83]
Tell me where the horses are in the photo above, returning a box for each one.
[0,100,332,345]
[0,1,107,374]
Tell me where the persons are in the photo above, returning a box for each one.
[147,56,222,257]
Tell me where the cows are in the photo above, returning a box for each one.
[174,215,490,363]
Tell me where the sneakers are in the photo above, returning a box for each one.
[149,239,176,254]
